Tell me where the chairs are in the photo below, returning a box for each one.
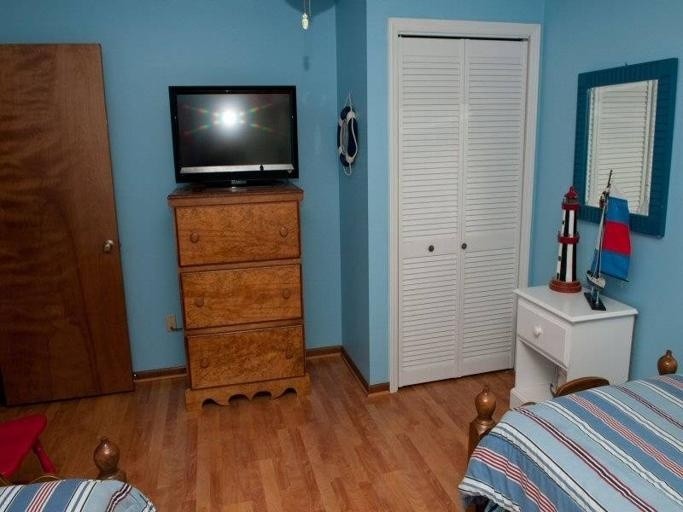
[0,413,62,486]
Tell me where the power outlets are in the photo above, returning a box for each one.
[166,313,178,333]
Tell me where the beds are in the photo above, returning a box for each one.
[457,349,682,512]
[0,436,158,512]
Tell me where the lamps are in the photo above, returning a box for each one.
[299,0,312,30]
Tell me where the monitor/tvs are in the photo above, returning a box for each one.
[167,84,300,189]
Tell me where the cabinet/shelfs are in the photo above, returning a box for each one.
[387,17,542,394]
[165,181,312,412]
[509,282,639,410]
